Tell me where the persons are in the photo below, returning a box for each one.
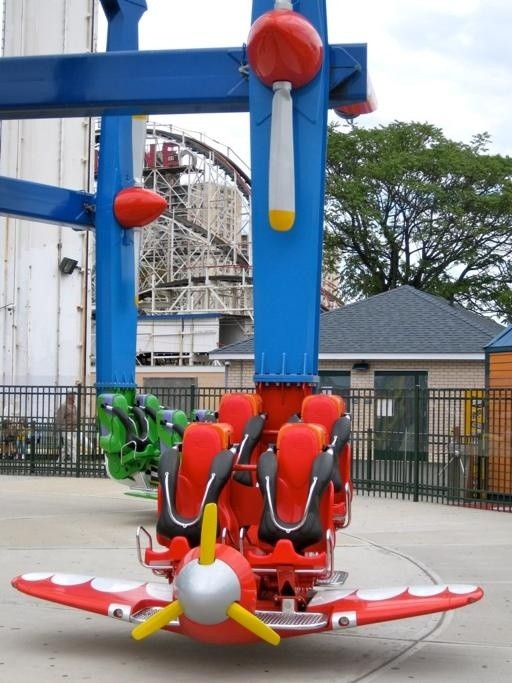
[52,391,93,469]
